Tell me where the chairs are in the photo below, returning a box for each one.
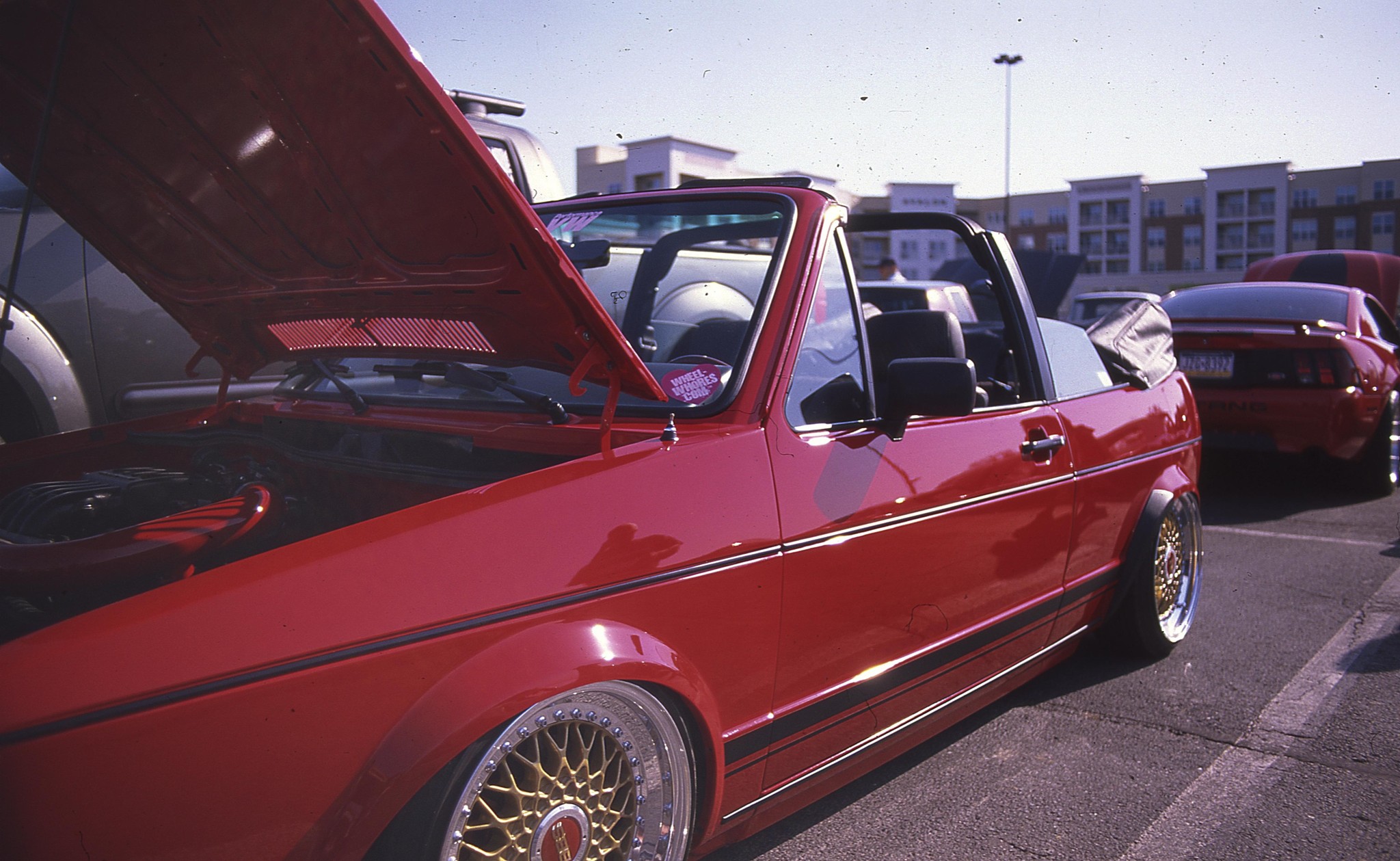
[866,309,965,416]
[883,357,978,417]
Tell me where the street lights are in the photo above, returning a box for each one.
[993,54,1023,238]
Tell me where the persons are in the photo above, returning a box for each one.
[877,258,908,281]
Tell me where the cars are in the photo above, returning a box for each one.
[0,1,1214,861]
[1156,248,1400,495]
[0,83,975,431]
[1064,291,1162,335]
